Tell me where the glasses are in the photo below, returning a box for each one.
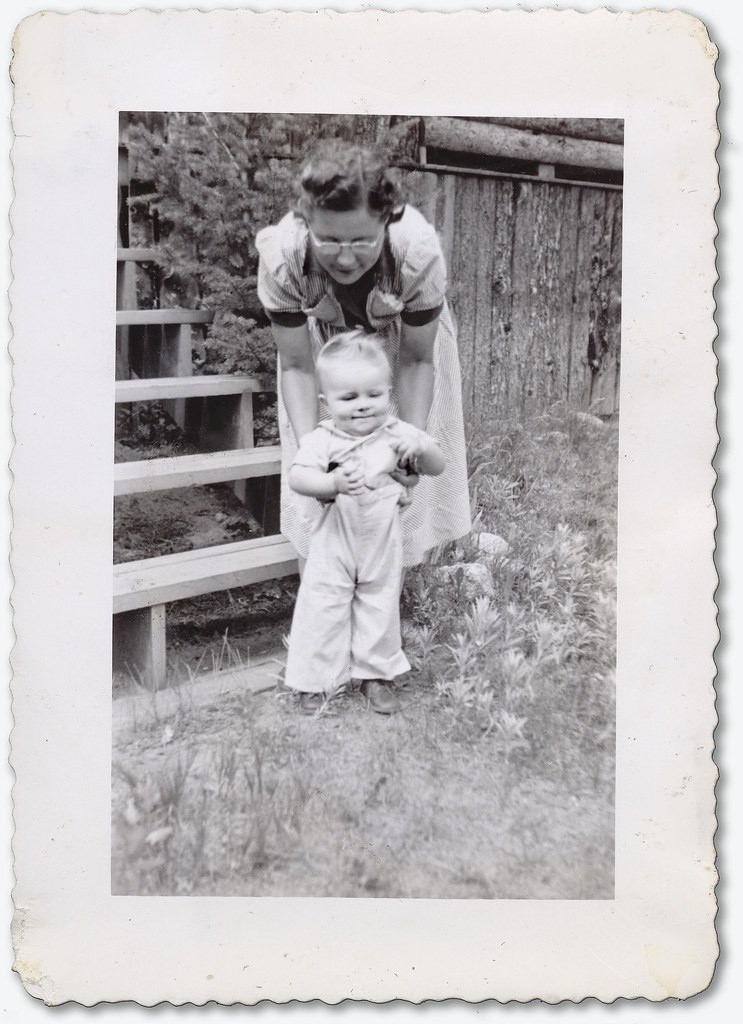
[307,220,385,254]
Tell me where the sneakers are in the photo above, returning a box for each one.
[301,693,327,716]
[360,680,399,713]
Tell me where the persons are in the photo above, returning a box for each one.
[277,329,448,716]
[253,137,473,637]
